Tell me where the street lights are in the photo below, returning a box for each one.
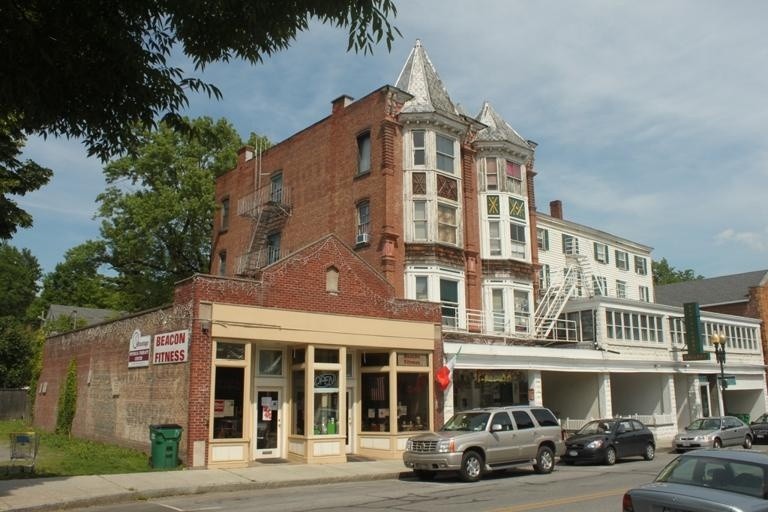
[711,332,727,416]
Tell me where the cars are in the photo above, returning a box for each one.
[257,417,270,446]
[559,418,656,465]
[622,449,768,512]
[454,372,500,411]
[313,408,340,427]
[670,415,752,452]
[748,411,767,445]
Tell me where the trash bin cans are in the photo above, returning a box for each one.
[149,424,184,469]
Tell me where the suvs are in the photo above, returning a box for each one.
[400,404,566,482]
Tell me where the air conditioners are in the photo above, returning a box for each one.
[355,234,368,245]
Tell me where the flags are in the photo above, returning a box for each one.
[435,354,456,389]
